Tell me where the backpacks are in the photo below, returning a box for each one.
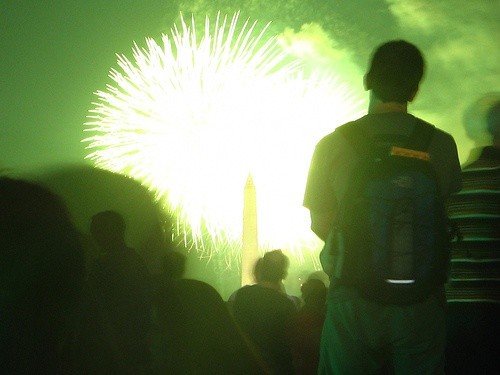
[339,117,453,305]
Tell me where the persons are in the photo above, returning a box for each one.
[448,100,500,375]
[1,175,77,375]
[160,251,185,279]
[234,249,298,374]
[87,211,147,375]
[298,279,326,375]
[305,40,462,375]
